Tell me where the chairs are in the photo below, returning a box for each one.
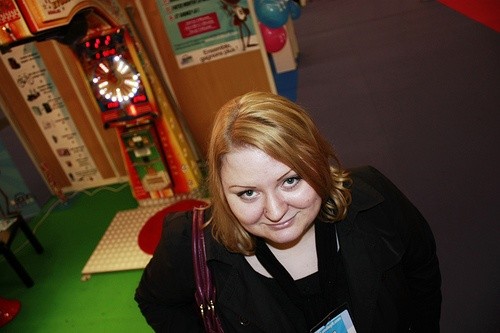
[0,188,45,289]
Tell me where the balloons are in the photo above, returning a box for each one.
[255,0,289,27]
[259,23,287,53]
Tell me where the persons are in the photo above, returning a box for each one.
[134,92,443,333]
